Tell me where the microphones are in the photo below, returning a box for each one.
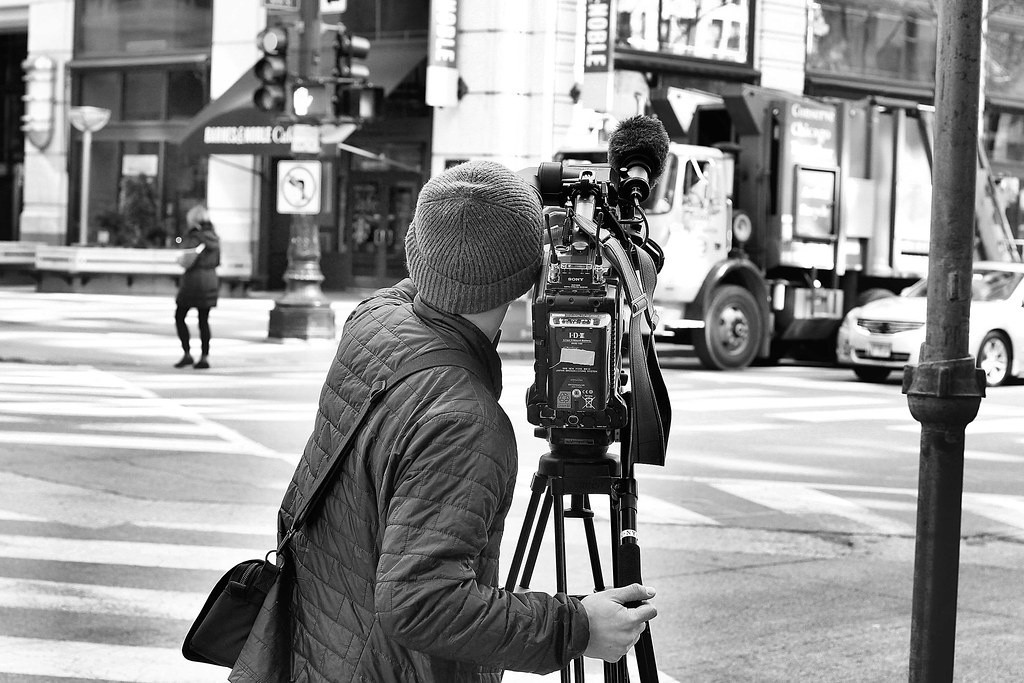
[607,115,670,203]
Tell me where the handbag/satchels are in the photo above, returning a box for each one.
[181,559,279,670]
[175,243,205,269]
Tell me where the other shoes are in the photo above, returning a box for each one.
[194,362,209,368]
[175,356,193,368]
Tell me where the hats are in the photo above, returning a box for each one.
[405,162,545,314]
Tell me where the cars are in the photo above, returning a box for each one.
[833,256,1023,386]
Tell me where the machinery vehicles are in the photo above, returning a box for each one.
[536,81,1024,371]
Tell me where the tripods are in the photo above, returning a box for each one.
[498,423,658,683]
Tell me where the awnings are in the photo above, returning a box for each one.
[174,37,432,176]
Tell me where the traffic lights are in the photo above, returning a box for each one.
[337,32,384,124]
[254,19,294,114]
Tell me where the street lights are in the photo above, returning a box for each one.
[62,104,115,250]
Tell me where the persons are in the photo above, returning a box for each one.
[173,205,220,368]
[227,158,656,683]
